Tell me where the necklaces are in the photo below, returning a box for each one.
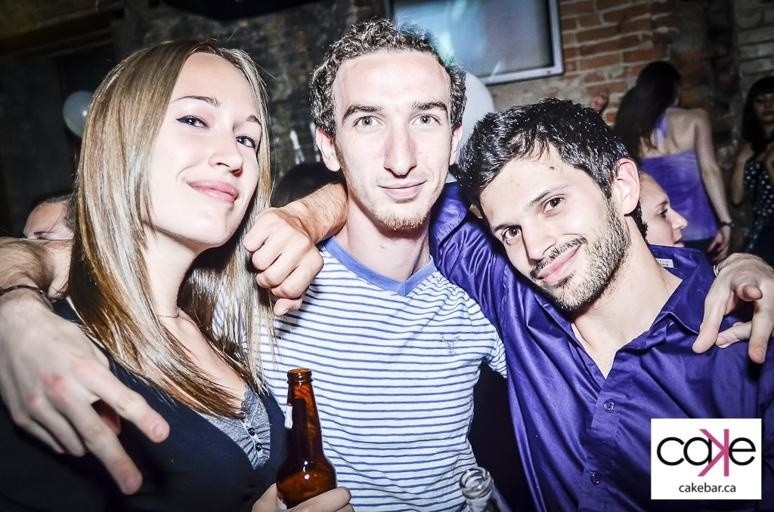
[154,308,185,320]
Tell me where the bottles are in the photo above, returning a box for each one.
[274,367,339,511]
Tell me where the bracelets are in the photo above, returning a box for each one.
[719,220,735,227]
[0,284,44,297]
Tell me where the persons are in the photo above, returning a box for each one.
[0,36,355,512]
[635,170,688,248]
[615,61,737,261]
[269,161,343,208]
[0,17,772,511]
[242,95,773,511]
[20,190,77,240]
[729,76,774,252]
